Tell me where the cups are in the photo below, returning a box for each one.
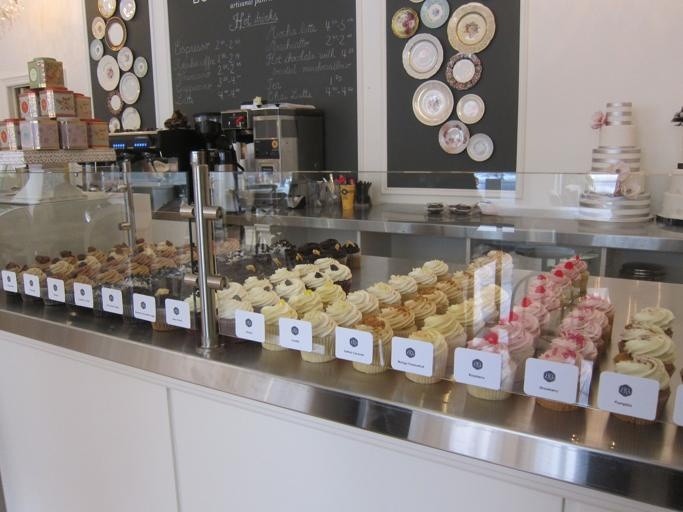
[339,184,354,209]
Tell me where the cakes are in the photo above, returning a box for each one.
[352,245,515,374]
[578,101,651,222]
[88,232,360,334]
[613,306,678,424]
[661,168,683,221]
[258,278,341,351]
[463,255,591,401]
[534,293,614,413]
[296,256,448,365]
[189,249,351,338]
[404,285,512,384]
[2,235,242,309]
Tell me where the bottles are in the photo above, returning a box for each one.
[190,150,213,206]
[210,149,242,213]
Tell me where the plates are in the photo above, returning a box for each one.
[446,1,494,53]
[88,0,148,135]
[411,77,453,127]
[437,121,471,155]
[425,201,473,215]
[420,0,450,28]
[465,132,494,163]
[455,94,486,123]
[443,52,483,88]
[401,33,443,80]
[391,7,419,40]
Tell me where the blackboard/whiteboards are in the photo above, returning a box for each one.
[84,0,523,206]
